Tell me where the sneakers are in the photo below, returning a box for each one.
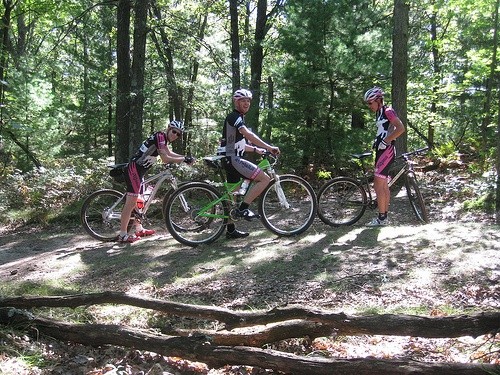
[118,234,140,243]
[231,207,261,222]
[135,229,156,237]
[225,230,250,240]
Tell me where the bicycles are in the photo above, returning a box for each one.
[316,146,433,225]
[165,148,316,248]
[83,154,217,234]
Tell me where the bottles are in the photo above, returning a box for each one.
[136,192,144,213]
[145,186,152,203]
[239,177,251,195]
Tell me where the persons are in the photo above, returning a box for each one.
[118,120,194,243]
[217,89,280,239]
[363,87,406,226]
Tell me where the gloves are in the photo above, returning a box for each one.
[184,155,195,165]
[378,140,388,150]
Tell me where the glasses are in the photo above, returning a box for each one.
[171,129,180,136]
[368,97,384,105]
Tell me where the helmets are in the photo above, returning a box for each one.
[168,120,184,132]
[233,89,253,101]
[364,88,384,103]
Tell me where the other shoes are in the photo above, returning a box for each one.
[365,217,389,227]
